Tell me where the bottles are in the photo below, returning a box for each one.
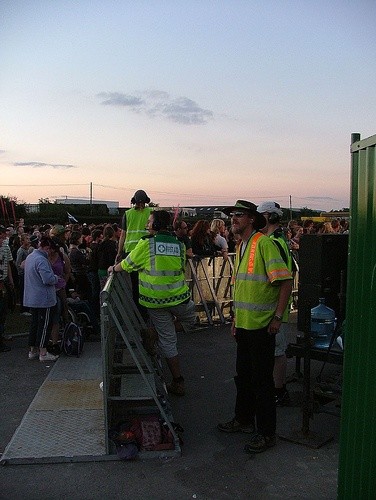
[310,297,336,349]
[68,289,74,298]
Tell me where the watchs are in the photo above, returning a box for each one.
[273,314,282,321]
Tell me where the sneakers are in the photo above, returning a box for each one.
[29,350,40,358]
[39,352,59,361]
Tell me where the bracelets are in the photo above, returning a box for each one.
[113,265,117,273]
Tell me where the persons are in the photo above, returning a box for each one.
[114,190,156,263]
[0,200,350,453]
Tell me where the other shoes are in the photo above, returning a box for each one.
[0,344,12,352]
[275,383,290,406]
[218,419,255,434]
[245,435,276,452]
[166,376,185,394]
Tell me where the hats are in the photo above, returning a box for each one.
[0,225,10,232]
[257,201,283,216]
[49,225,70,237]
[222,199,267,229]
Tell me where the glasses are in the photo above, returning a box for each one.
[183,226,189,229]
[230,212,244,218]
